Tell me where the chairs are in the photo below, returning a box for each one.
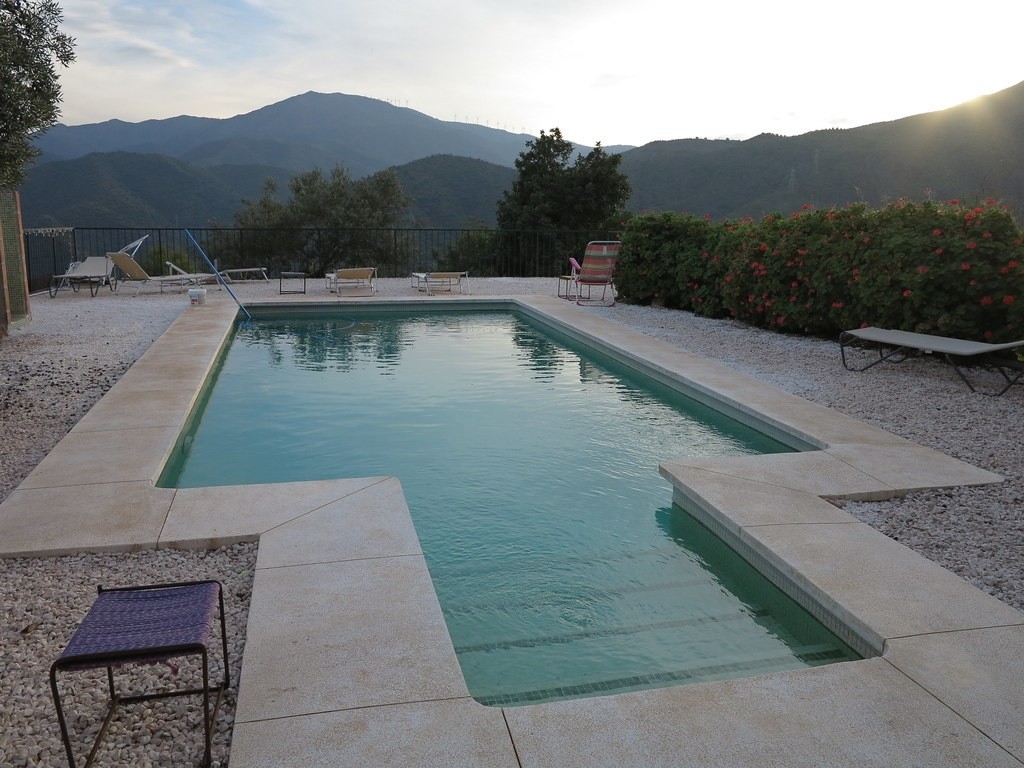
[567,240,622,307]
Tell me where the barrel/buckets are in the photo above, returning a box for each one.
[188,287,208,305]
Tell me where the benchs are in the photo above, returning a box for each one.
[839,326,1024,398]
[325,267,379,297]
[48,234,270,298]
[411,271,471,297]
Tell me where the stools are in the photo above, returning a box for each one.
[49,579,230,768]
[280,272,306,295]
[558,276,590,300]
